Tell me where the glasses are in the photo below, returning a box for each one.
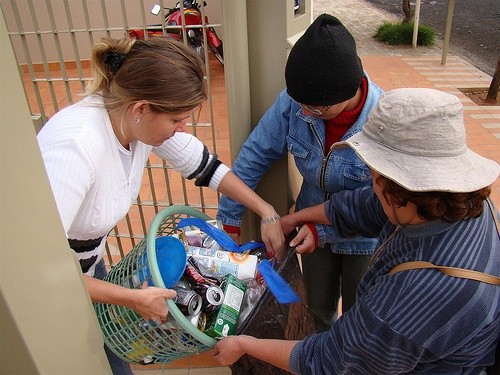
[288,96,329,115]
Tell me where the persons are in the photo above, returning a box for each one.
[212,87,500,374]
[216,13,383,331]
[37,37,285,375]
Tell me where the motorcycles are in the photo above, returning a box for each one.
[124,0,225,68]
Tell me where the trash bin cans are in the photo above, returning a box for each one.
[95,203,250,366]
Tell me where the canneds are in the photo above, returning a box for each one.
[108,218,223,334]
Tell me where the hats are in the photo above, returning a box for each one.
[284,14,364,106]
[330,87,500,191]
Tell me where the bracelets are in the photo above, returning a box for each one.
[261,215,281,223]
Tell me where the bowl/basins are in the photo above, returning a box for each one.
[138,236,187,289]
